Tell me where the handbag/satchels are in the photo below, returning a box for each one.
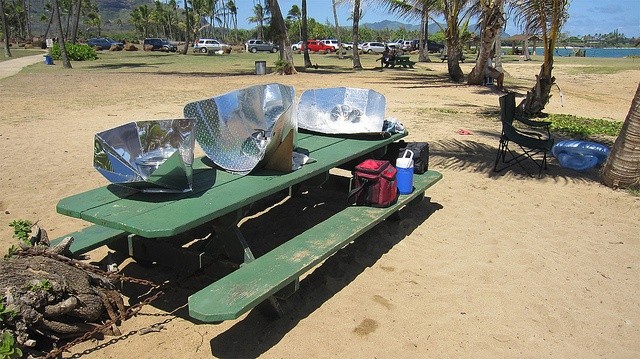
[347,159,398,207]
[388,140,429,175]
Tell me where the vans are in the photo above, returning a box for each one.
[404,40,412,49]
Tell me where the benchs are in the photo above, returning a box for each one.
[188,170,442,323]
[49,224,128,257]
[386,61,416,69]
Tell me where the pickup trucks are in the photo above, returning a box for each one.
[389,39,405,49]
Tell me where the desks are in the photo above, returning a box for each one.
[397,55,412,61]
[56,130,408,320]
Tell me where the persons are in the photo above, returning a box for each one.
[376,44,388,69]
[389,46,397,68]
[194,38,199,52]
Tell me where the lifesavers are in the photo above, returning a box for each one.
[552,141,609,170]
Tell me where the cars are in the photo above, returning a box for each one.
[318,40,342,51]
[194,39,228,53]
[292,40,303,51]
[144,38,178,52]
[340,42,361,50]
[361,42,390,53]
[85,38,125,51]
[245,39,280,52]
[302,40,335,54]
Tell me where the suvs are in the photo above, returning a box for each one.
[412,39,445,53]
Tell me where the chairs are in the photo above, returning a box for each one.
[493,93,554,178]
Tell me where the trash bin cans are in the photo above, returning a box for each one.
[43,55,51,65]
[255,61,266,75]
